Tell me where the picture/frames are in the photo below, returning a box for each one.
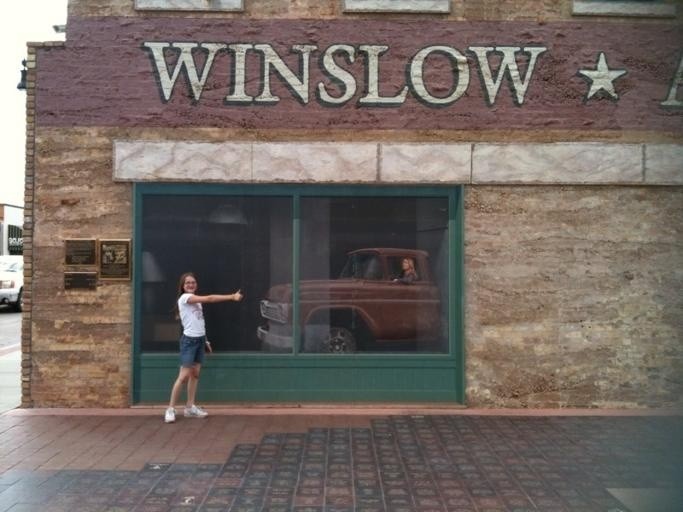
[63,238,132,293]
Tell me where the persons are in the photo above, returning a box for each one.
[390,255,419,285]
[161,271,244,425]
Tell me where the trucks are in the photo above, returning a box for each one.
[257,248,441,352]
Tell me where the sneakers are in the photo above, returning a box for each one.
[183,405,208,419]
[165,407,177,423]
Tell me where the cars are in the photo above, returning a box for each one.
[0,255,22,311]
